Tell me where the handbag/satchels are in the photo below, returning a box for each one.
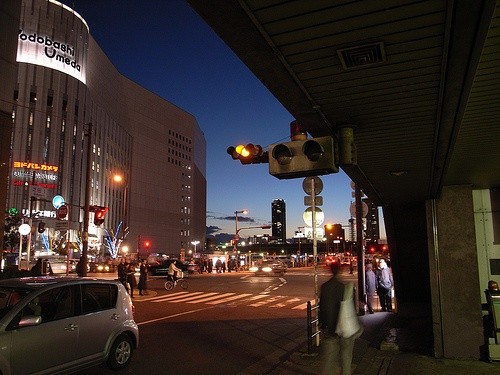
[336,283,362,338]
[126,283,131,290]
[176,271,183,278]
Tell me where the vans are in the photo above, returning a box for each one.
[64,259,90,273]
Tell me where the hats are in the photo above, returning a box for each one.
[378,259,384,262]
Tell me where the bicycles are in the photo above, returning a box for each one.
[164,270,189,291]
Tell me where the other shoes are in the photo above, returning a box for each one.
[388,309,392,312]
[369,309,374,314]
[139,293,144,296]
[380,307,386,312]
[130,294,135,298]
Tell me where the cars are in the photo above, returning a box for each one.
[89,262,110,273]
[326,257,335,266]
[148,259,194,277]
[254,260,287,276]
[0,276,139,375]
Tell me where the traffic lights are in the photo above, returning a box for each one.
[267,134,335,174]
[38,222,46,234]
[95,207,107,227]
[226,143,264,162]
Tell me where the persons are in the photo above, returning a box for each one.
[308,256,312,266]
[76,257,88,277]
[349,259,353,274]
[137,258,150,296]
[182,259,194,265]
[35,258,43,275]
[89,258,96,273]
[365,255,393,314]
[97,253,117,273]
[319,261,356,375]
[486,280,500,303]
[338,257,340,263]
[198,257,241,274]
[117,258,137,298]
[317,257,320,265]
[168,260,181,289]
[153,260,159,265]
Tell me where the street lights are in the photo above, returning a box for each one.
[235,209,248,270]
[114,175,127,244]
